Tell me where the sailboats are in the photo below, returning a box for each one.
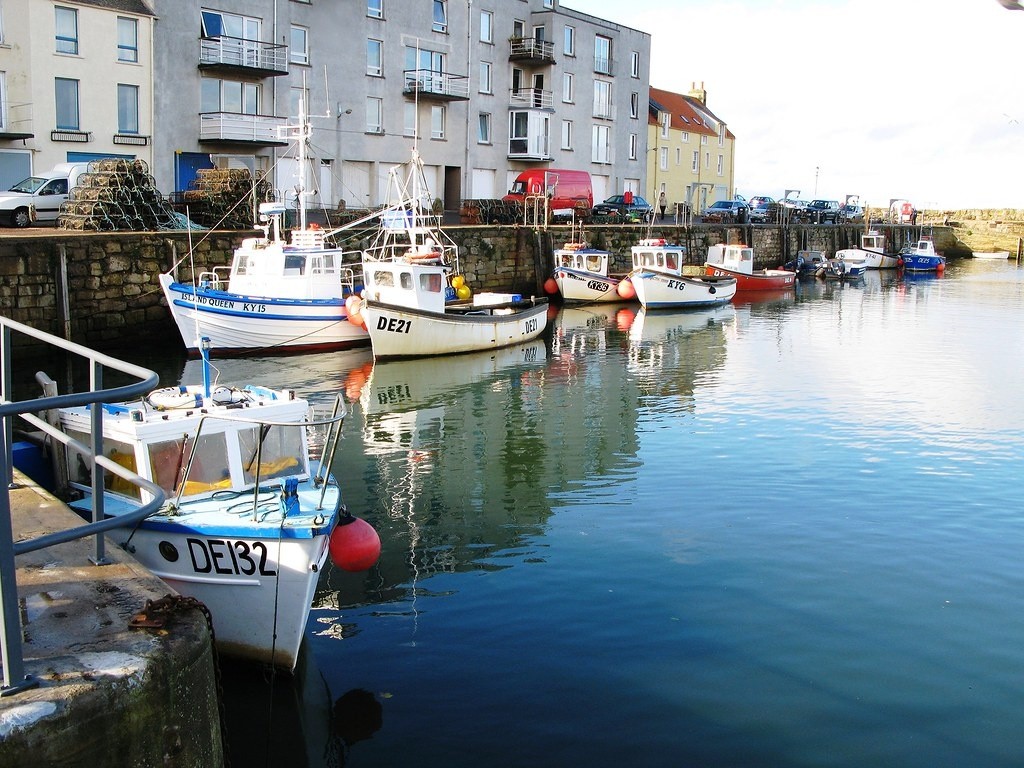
[351,37,551,359]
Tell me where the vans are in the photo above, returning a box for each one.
[502,170,595,216]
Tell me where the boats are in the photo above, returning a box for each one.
[629,191,737,310]
[777,250,866,280]
[352,339,548,423]
[8,337,379,673]
[704,231,796,291]
[833,220,902,270]
[624,303,737,349]
[899,213,947,273]
[554,301,639,335]
[553,209,638,302]
[158,92,372,358]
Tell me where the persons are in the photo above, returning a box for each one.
[910,209,918,225]
[656,192,672,220]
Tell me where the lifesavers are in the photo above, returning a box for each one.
[149,382,231,411]
[563,243,586,251]
[639,238,668,246]
[404,252,441,264]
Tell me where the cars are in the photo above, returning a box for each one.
[748,202,788,224]
[778,199,811,216]
[701,200,751,222]
[591,194,654,225]
[838,205,865,224]
[750,196,775,210]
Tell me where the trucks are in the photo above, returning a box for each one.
[0,162,103,227]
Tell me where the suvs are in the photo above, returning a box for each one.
[803,199,842,227]
